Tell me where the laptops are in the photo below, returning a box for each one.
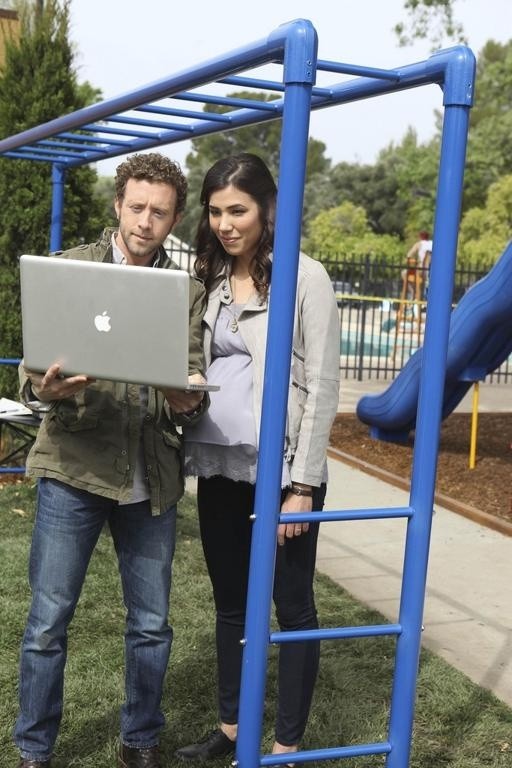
[17,252,224,395]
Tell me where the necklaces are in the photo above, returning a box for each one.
[230,263,257,333]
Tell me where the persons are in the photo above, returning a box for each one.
[13,152,211,768]
[400,230,432,290]
[173,152,340,768]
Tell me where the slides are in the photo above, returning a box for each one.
[357,242,512,442]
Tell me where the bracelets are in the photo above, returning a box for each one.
[285,485,316,502]
[181,400,207,421]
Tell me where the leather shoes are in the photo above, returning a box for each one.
[175,728,236,758]
[119,743,161,768]
[21,756,51,768]
